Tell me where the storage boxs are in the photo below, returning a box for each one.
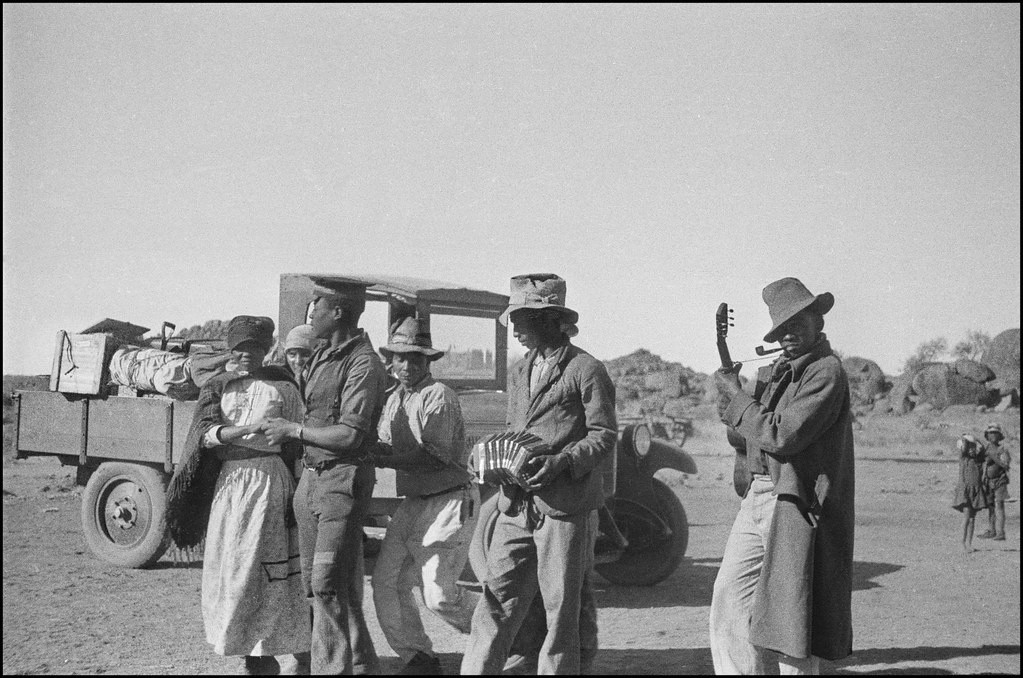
[50,319,151,397]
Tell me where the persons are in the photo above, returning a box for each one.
[710,276,854,676]
[458,274,618,676]
[978,424,1012,540]
[375,317,486,676]
[951,433,988,553]
[261,274,390,676]
[197,315,306,676]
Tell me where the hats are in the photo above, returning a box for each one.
[497,272,579,328]
[283,323,322,354]
[379,316,446,362]
[984,422,1005,442]
[957,434,977,451]
[308,272,377,314]
[761,277,834,343]
[226,315,275,356]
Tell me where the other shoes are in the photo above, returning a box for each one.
[295,659,311,674]
[245,658,281,675]
[957,547,980,554]
[976,529,1006,541]
[533,617,551,658]
[396,652,444,675]
[353,659,381,675]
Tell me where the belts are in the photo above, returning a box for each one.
[307,459,338,472]
[419,484,467,501]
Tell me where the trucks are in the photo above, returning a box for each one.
[17,271,696,592]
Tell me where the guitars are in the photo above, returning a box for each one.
[715,303,750,499]
